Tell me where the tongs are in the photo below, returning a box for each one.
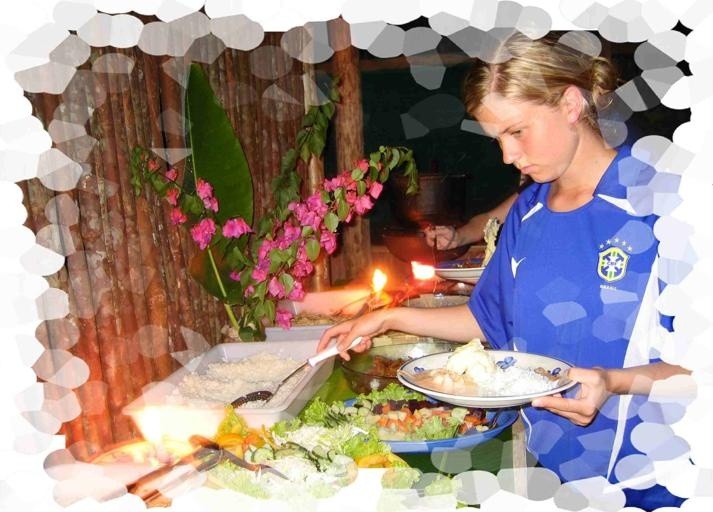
[100,442,222,504]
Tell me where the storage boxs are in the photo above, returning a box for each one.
[118,335,336,457]
[258,288,372,342]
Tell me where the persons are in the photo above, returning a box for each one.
[316,24,688,512]
[422,176,534,252]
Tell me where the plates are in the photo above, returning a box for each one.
[397,350,581,410]
[336,392,521,454]
[434,268,484,284]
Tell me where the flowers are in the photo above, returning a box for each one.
[127,58,420,342]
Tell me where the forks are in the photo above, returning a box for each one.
[186,435,290,485]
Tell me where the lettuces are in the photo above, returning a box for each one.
[204,382,461,501]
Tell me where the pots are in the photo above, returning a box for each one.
[367,216,471,266]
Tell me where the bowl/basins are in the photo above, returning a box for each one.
[340,340,454,398]
[400,292,471,313]
